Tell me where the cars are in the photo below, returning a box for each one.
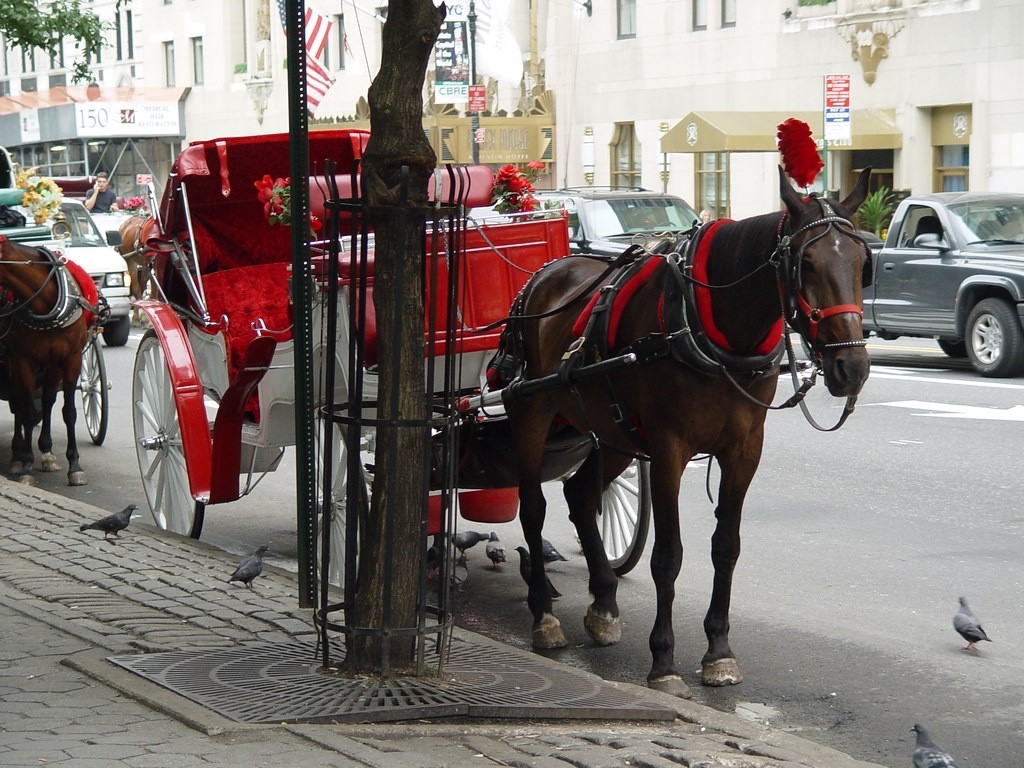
[0,198,132,345]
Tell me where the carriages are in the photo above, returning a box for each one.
[39,176,158,327]
[0,235,113,488]
[132,118,884,702]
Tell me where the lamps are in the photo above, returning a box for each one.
[835,13,905,86]
[582,127,595,185]
[659,122,670,193]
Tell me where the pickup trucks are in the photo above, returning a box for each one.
[855,191,1024,377]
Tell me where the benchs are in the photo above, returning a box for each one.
[77,197,126,209]
[174,129,569,423]
[51,178,90,197]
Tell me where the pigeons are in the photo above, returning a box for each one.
[910,721,962,768]
[953,596,993,650]
[427,531,568,598]
[227,544,269,591]
[79,505,139,538]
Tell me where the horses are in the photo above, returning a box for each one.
[115,215,160,329]
[1,233,86,486]
[497,164,875,693]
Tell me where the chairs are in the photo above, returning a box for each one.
[908,215,944,248]
[977,219,1002,240]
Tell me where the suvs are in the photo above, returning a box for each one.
[464,184,705,260]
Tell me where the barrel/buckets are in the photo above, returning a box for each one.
[425,495,446,536]
[461,486,519,523]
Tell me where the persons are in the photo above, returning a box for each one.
[85,172,119,214]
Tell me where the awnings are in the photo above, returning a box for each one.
[660,110,902,154]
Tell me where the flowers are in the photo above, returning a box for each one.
[123,195,151,214]
[492,159,569,222]
[777,116,826,197]
[16,165,63,224]
[255,174,323,232]
